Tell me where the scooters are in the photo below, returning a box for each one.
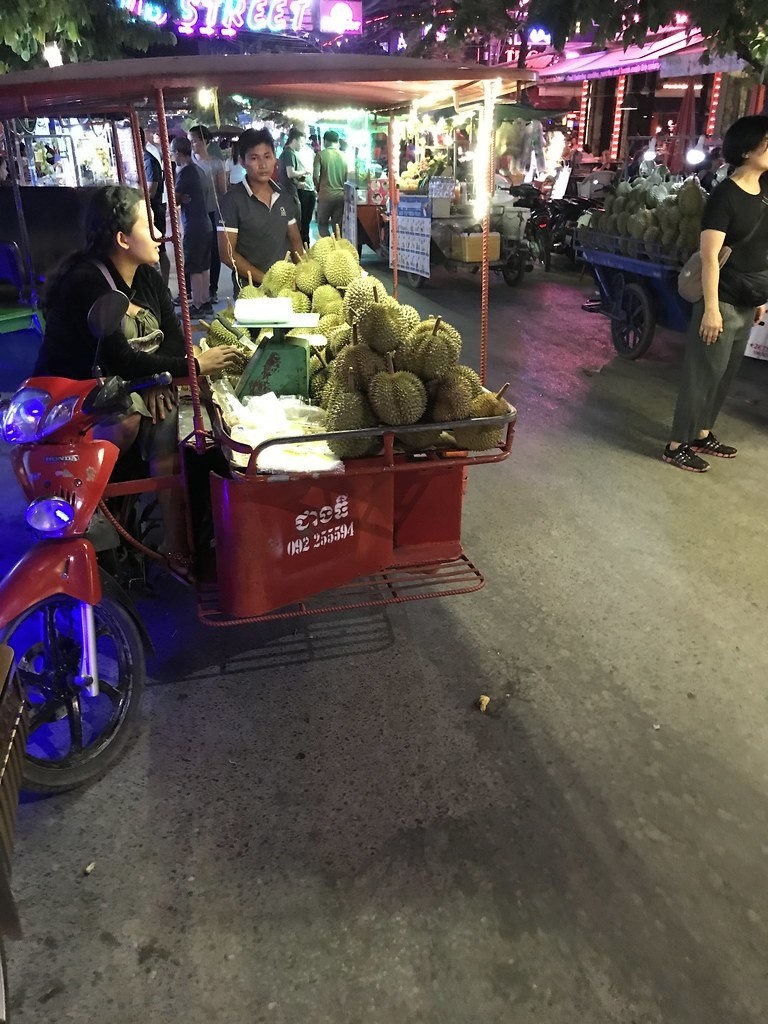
[500,182,621,268]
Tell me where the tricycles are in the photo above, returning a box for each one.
[0,54,539,791]
[378,203,530,290]
[573,225,768,364]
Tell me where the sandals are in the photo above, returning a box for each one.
[156,545,198,586]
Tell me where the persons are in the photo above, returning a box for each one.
[44,145,55,165]
[20,141,32,183]
[570,136,724,194]
[661,114,768,473]
[135,124,347,300]
[0,156,9,181]
[30,179,247,594]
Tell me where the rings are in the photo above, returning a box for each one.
[158,395,165,399]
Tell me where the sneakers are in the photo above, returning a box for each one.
[178,301,214,319]
[210,297,220,306]
[172,294,193,305]
[690,431,738,458]
[662,443,710,472]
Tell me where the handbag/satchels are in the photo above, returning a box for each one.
[678,245,732,302]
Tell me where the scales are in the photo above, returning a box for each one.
[232,312,322,398]
